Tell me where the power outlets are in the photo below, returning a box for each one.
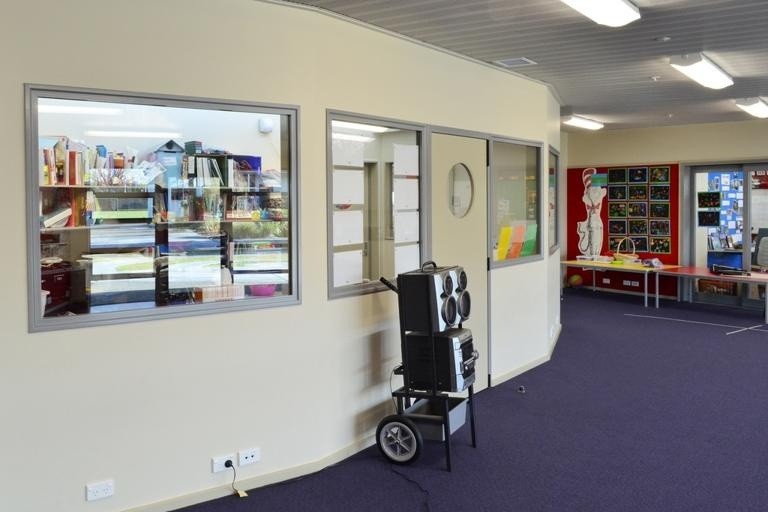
[211,447,261,472]
[84,477,115,502]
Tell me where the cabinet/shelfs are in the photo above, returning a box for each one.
[41,183,289,320]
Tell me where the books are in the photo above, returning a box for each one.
[42,146,135,187]
[42,207,73,228]
[188,156,248,188]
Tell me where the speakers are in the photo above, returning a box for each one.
[398,261,479,393]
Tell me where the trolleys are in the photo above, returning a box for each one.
[372,262,483,477]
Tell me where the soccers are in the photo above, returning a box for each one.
[570,274,582,288]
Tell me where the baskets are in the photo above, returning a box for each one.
[615,237,639,260]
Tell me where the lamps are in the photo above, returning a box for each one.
[669,54,768,123]
[561,115,605,131]
[560,0,641,29]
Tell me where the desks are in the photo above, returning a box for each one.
[559,260,767,324]
